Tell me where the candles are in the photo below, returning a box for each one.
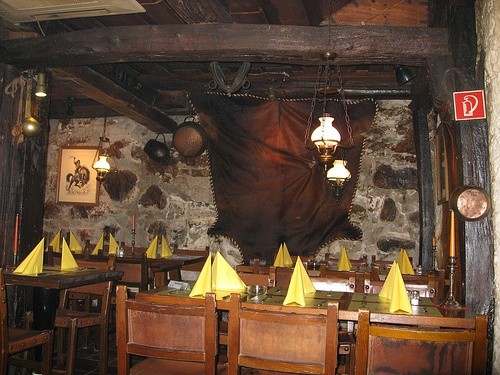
[15,214,19,254]
[433,237,435,245]
[449,212,455,256]
[132,214,135,229]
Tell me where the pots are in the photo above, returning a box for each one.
[173,116,208,165]
[449,159,491,222]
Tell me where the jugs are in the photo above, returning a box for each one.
[144,131,170,164]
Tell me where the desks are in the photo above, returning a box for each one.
[3,243,478,375]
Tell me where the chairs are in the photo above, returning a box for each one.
[0,254,490,375]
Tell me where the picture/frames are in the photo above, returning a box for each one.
[55,146,100,206]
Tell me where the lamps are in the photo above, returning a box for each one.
[145,134,170,161]
[92,113,115,182]
[304,0,355,202]
[34,73,47,98]
[395,64,415,85]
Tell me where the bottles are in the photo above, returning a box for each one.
[116,247,125,257]
[416,265,423,275]
[407,290,420,306]
[307,258,317,268]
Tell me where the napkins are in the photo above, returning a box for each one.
[12,229,419,314]
